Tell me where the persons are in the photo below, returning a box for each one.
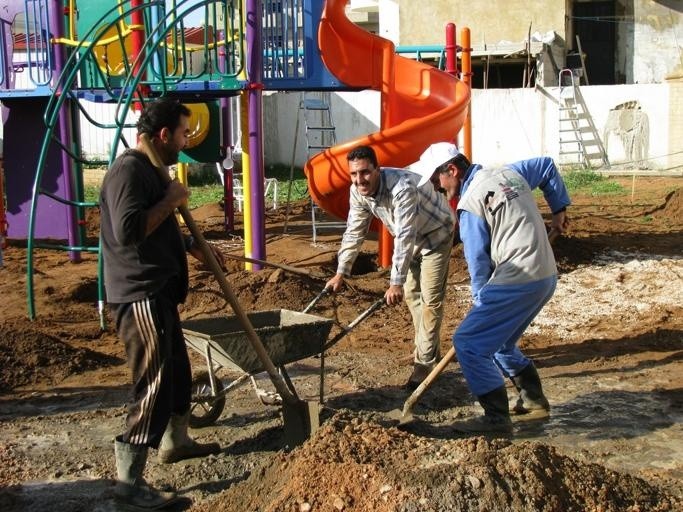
[323,145,457,390]
[96,95,229,512]
[414,142,572,432]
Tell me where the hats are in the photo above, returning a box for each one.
[415,141,459,189]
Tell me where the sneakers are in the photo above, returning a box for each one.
[406,364,434,388]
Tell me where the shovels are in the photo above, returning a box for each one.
[382,214,569,436]
[138,131,320,444]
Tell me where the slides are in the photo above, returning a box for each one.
[304,1,472,266]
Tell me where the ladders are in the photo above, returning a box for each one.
[283,91,338,244]
[556,67,611,174]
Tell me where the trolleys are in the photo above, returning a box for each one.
[180,282,393,432]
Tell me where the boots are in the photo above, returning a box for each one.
[508,358,552,423]
[158,404,220,465]
[113,433,178,511]
[451,383,515,433]
